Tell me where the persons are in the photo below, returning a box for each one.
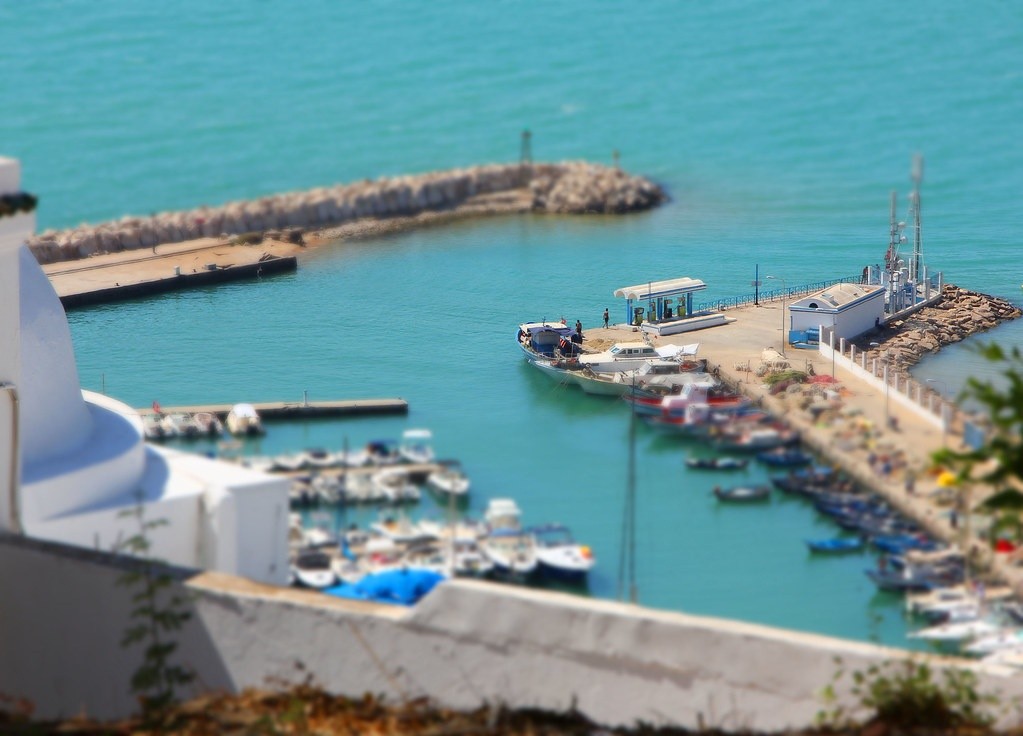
[561,308,609,338]
[863,250,892,282]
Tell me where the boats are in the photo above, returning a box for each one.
[620,380,1023,661]
[514,320,587,364]
[569,361,705,395]
[137,400,265,440]
[527,341,688,386]
[221,429,592,591]
